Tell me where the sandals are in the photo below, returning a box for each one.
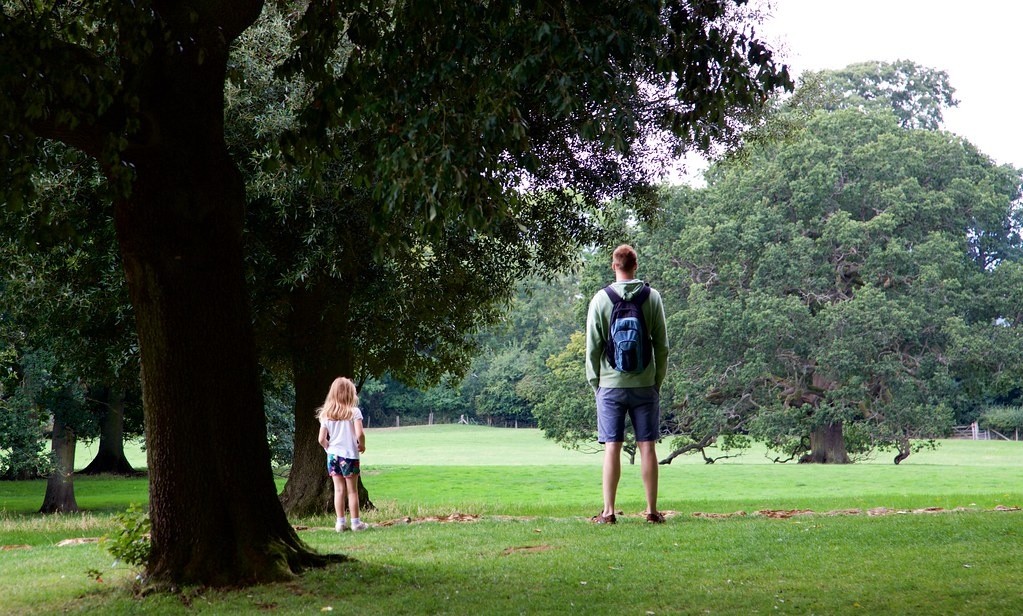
[647,513,665,524]
[592,511,617,525]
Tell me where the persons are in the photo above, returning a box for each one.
[585,243,670,524]
[314,376,370,533]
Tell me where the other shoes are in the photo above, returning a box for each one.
[351,522,368,532]
[335,523,348,533]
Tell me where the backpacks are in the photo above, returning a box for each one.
[602,283,653,374]
